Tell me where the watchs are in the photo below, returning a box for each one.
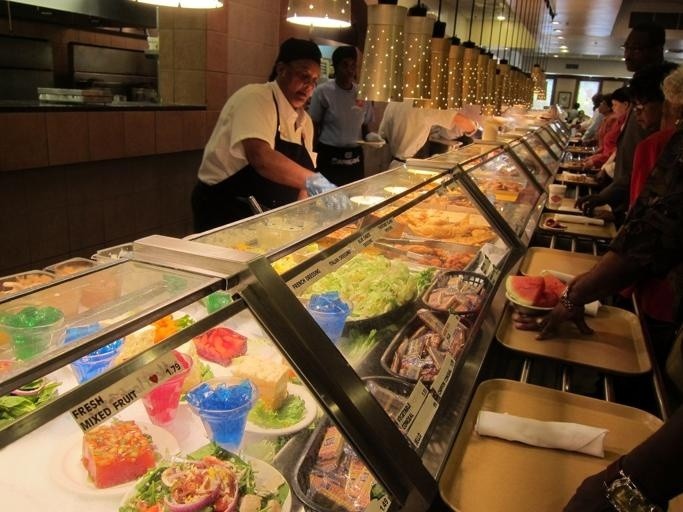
[602,455,668,512]
[559,285,582,313]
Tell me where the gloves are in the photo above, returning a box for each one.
[366,131,385,149]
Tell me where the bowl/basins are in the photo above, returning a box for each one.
[506,291,557,312]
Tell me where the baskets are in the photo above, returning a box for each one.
[381,309,473,385]
[421,269,491,319]
[289,375,415,512]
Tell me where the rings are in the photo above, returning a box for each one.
[536,318,544,329]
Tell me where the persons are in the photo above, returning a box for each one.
[511,124,683,372]
[310,46,380,187]
[189,38,323,235]
[630,74,665,139]
[575,20,669,220]
[561,413,683,512]
[377,99,482,167]
[628,63,683,372]
[563,85,633,182]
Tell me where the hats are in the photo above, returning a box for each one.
[269,38,321,81]
[591,93,603,111]
[332,45,357,65]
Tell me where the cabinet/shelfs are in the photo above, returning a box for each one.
[0,112,573,512]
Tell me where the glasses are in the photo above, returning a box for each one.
[633,99,649,113]
[285,63,319,89]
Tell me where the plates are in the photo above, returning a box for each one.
[246,378,317,435]
[53,422,179,500]
[351,195,387,211]
[358,140,387,148]
[383,186,427,196]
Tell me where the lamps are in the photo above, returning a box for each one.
[356,0,547,115]
[286,0,351,28]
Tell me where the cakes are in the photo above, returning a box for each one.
[193,328,247,367]
[179,361,214,402]
[230,356,305,427]
[81,417,155,489]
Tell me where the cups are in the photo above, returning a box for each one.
[57,322,123,384]
[2,306,63,361]
[142,352,191,427]
[502,117,516,141]
[549,184,566,208]
[571,128,576,138]
[482,119,497,141]
[186,377,259,453]
[310,292,353,347]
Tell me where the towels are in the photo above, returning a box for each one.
[553,214,605,226]
[474,410,609,458]
[583,300,602,316]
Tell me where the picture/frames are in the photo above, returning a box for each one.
[558,92,571,109]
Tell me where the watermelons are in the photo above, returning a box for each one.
[507,271,567,306]
